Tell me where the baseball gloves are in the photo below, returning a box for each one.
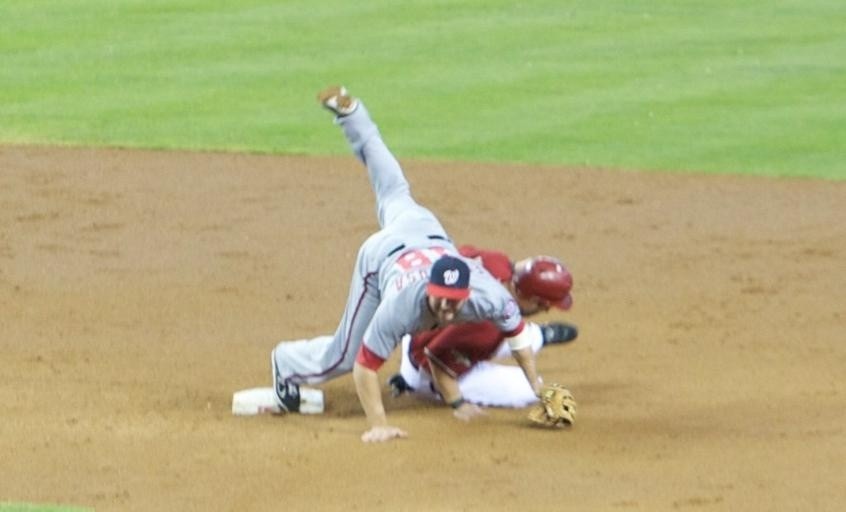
[528,385,576,429]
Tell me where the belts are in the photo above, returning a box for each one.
[388,233,447,255]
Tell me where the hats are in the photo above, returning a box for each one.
[426,254,471,301]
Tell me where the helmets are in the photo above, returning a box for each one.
[512,255,574,312]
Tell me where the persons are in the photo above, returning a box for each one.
[270,84,574,443]
[385,245,578,407]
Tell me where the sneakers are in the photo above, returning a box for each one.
[539,322,578,343]
[271,350,301,412]
[389,375,416,397]
[316,85,358,119]
